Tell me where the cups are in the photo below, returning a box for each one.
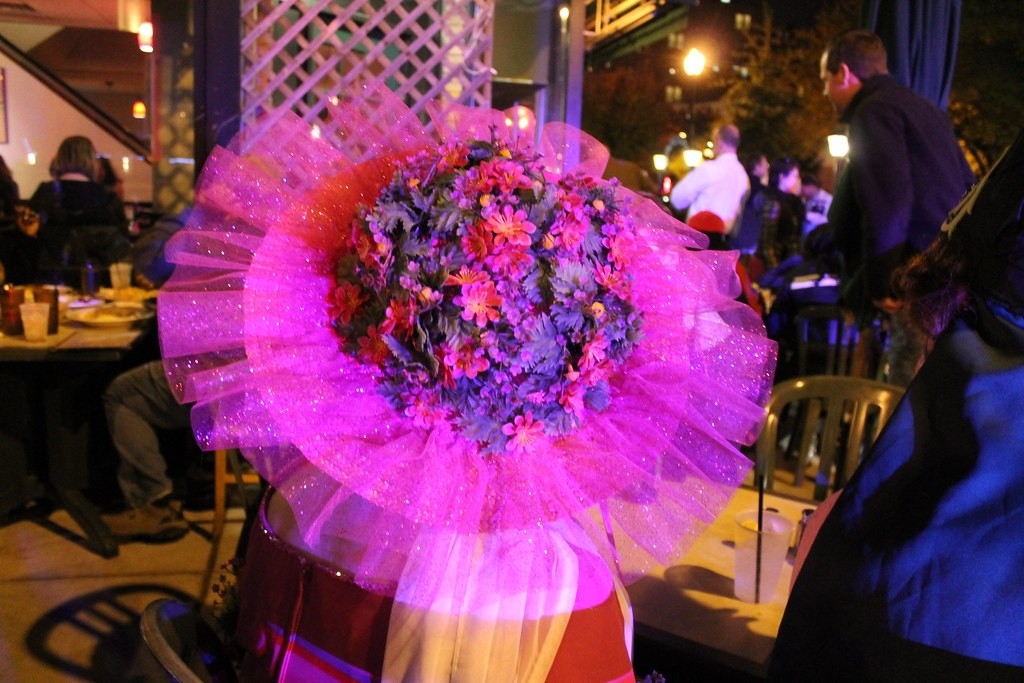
[108,262,132,289]
[33,287,58,334]
[0,286,26,336]
[733,511,793,605]
[81,263,102,298]
[19,303,50,342]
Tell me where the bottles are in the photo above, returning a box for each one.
[794,509,815,555]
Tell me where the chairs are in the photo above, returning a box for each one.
[211,408,260,546]
[752,377,908,500]
[137,597,232,682]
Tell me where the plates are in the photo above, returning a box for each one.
[65,304,155,328]
[16,283,159,307]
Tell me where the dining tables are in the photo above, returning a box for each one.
[0,293,154,559]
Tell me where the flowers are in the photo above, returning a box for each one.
[329,136,642,456]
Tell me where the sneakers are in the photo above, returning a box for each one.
[102,498,187,542]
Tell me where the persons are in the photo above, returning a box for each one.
[159,78,780,683]
[670,126,890,350]
[95,361,196,540]
[772,131,1024,683]
[802,31,979,470]
[0,137,135,291]
